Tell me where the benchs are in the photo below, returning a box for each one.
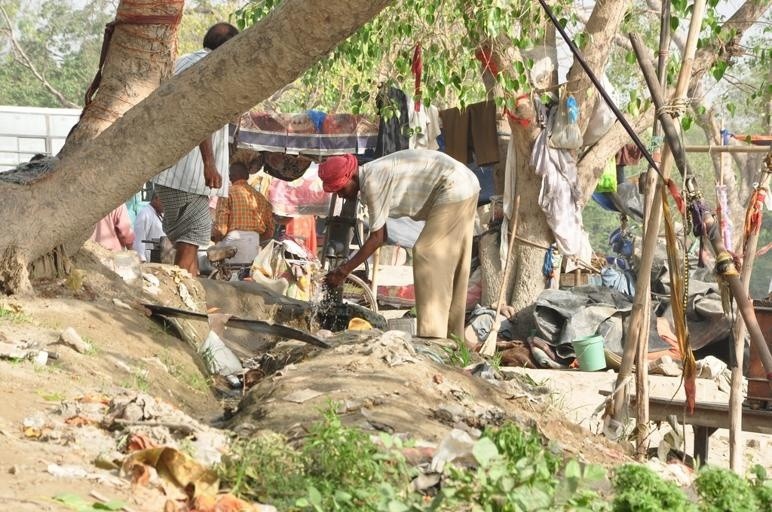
[599,389,772,471]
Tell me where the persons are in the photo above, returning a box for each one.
[91,201,135,254]
[132,192,165,263]
[145,22,239,275]
[544,242,555,290]
[610,214,632,267]
[212,161,274,279]
[318,148,481,342]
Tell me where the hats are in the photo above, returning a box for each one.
[318,154,357,192]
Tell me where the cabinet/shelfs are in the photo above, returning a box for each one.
[746,307,772,410]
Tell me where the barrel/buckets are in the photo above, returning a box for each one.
[571,334,607,372]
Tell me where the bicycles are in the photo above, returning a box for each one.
[208,239,379,313]
